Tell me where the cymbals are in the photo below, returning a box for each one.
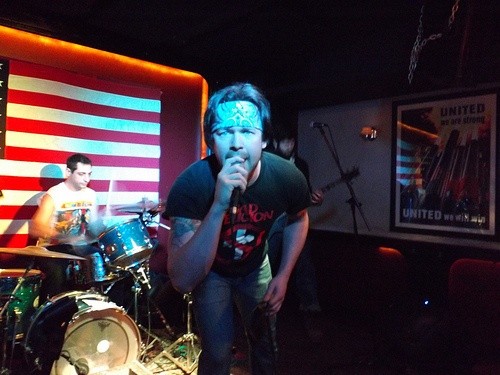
[0,245,88,261]
[116,202,164,214]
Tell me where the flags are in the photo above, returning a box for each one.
[0,57,161,271]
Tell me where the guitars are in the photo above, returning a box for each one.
[266,167,363,240]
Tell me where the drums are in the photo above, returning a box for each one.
[21,290,141,375]
[71,252,126,287]
[97,217,153,271]
[0,269,43,346]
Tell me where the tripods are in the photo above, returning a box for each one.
[144,292,200,375]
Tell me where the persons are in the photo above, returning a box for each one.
[164,82,312,375]
[29,153,97,305]
[271,126,324,312]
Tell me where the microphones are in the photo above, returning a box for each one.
[74,358,89,375]
[309,121,327,128]
[230,157,243,214]
